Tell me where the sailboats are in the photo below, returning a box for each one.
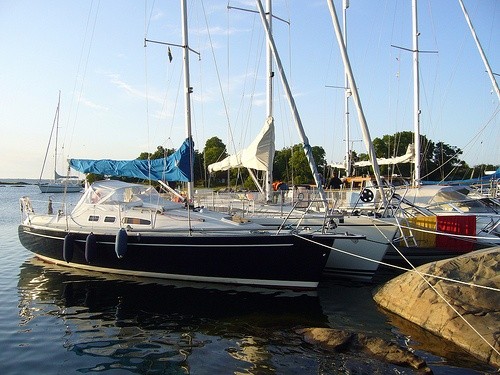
[138,0,500,282]
[17,0,368,290]
[33,90,82,193]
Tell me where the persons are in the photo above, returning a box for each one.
[325,170,343,191]
